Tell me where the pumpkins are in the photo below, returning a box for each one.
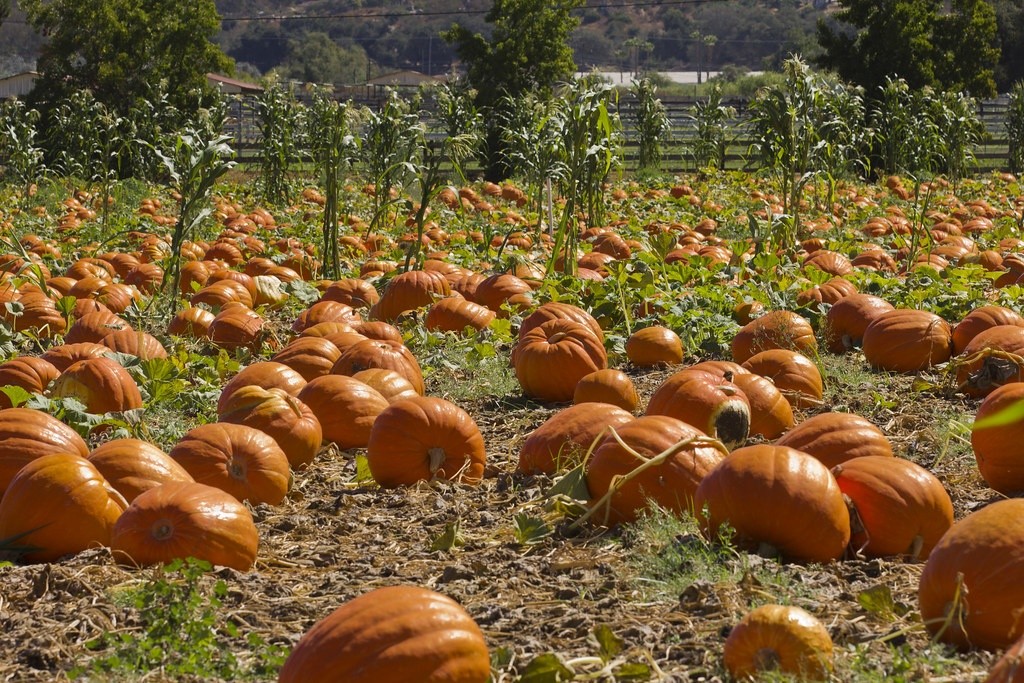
[0,173,1024,683]
